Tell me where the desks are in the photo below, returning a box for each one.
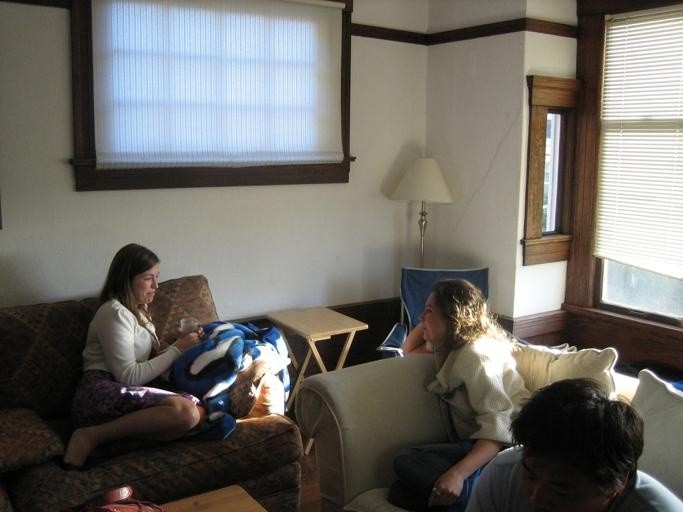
[263,299,369,459]
[136,482,271,512]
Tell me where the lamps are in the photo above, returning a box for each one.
[386,156,455,268]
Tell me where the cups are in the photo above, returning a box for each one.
[181,317,200,336]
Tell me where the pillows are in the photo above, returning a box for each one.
[618,362,682,502]
[507,337,621,403]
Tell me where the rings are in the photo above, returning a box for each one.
[432,488,440,498]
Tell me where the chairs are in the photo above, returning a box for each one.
[372,261,493,364]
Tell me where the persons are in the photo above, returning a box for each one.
[465,379,683,512]
[388,280,533,512]
[64,243,207,464]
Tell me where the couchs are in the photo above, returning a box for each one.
[0,272,304,512]
[287,337,682,510]
